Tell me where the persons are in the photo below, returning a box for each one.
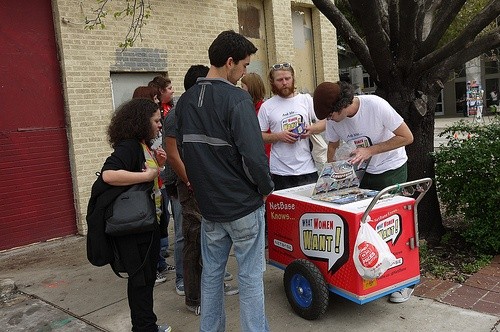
[101,98,172,332]
[131,65,271,316]
[313,81,416,302]
[258,62,326,191]
[176,30,276,332]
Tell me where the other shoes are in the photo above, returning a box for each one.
[187,305,201,315]
[176,277,185,296]
[163,264,176,273]
[224,271,233,281]
[155,270,166,282]
[155,320,172,332]
[223,283,240,295]
[164,252,170,258]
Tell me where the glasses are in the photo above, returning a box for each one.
[270,63,291,72]
[327,112,334,120]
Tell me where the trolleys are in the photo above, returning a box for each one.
[265,178,433,321]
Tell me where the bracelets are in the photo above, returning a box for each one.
[158,166,165,171]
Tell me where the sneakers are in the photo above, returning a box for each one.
[390,289,414,302]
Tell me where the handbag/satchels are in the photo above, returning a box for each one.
[304,93,329,164]
[104,137,158,233]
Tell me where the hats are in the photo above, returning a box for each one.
[314,82,340,120]
